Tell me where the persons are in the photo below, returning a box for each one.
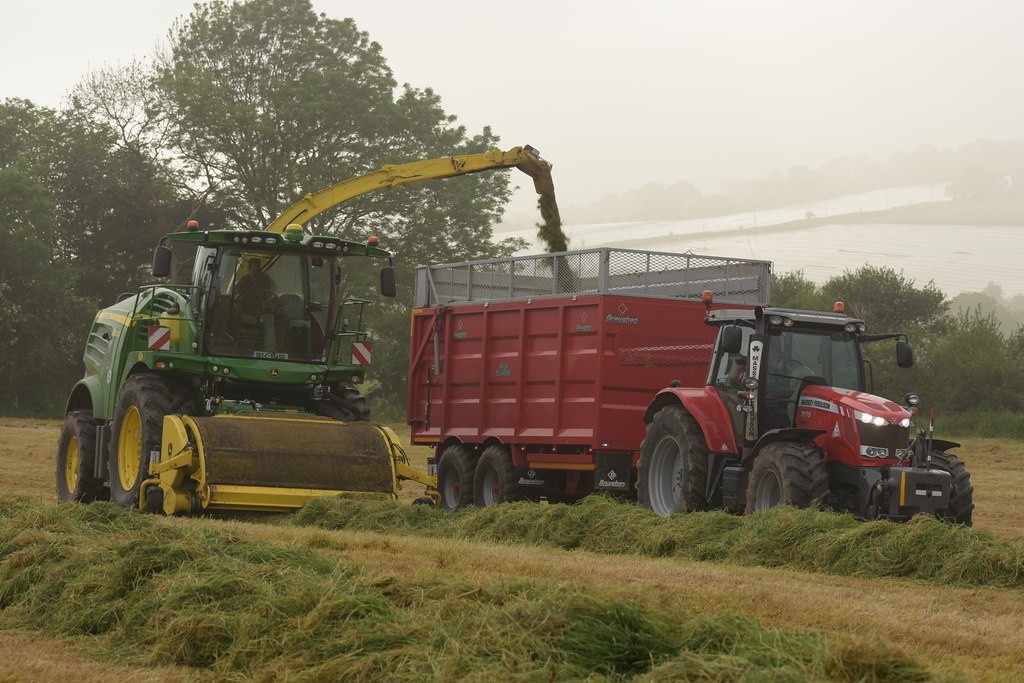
[229,258,282,359]
[766,340,800,398]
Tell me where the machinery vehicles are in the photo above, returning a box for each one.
[412,248,975,524]
[53,147,575,524]
